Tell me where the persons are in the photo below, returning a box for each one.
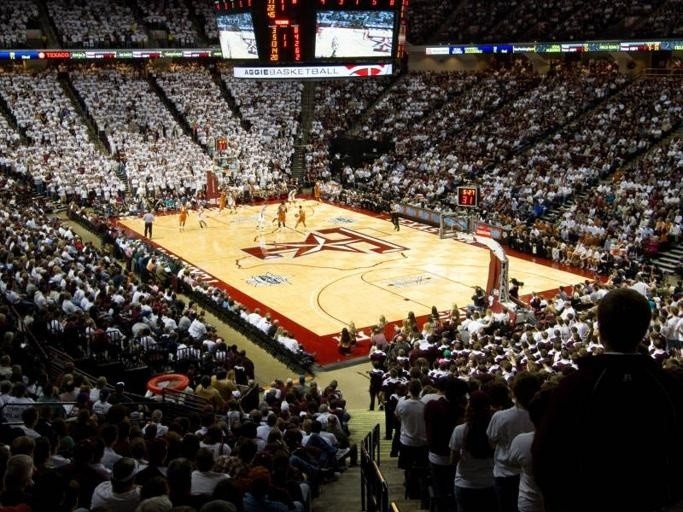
[0,0,681,512]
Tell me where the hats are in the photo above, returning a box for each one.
[110,456,147,484]
[63,362,75,368]
[114,381,126,389]
[469,390,490,408]
